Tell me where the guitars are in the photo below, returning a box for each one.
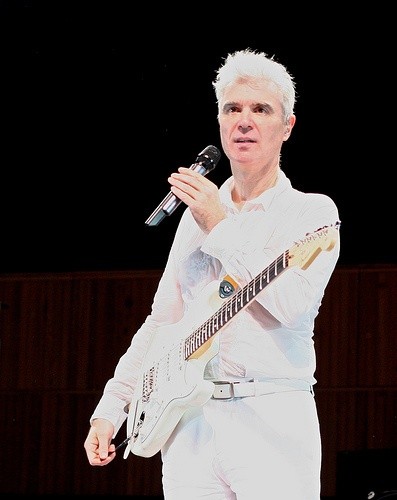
[126,220,342,458]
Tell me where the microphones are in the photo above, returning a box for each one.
[144,145,221,227]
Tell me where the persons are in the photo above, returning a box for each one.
[84,49,341,500]
